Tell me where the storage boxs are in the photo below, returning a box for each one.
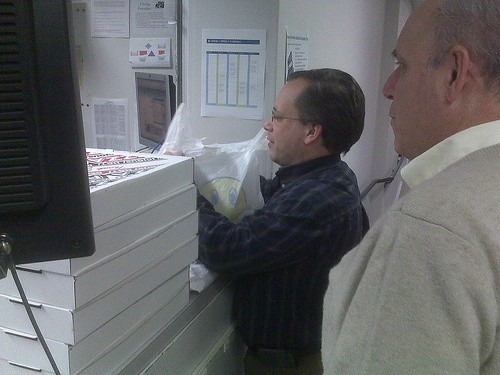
[0,148,199,375]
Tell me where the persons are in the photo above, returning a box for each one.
[322,0,500,375]
[196,68,371,375]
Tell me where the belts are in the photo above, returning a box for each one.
[246,345,320,357]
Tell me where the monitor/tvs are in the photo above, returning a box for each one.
[0,0,95,280]
[135,72,176,150]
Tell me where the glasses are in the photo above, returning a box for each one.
[271,111,327,139]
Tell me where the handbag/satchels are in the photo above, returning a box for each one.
[157,103,275,225]
